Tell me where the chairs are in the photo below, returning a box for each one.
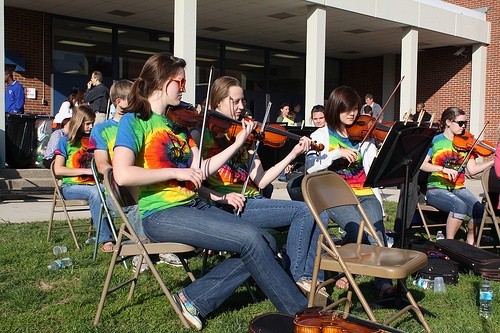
[46,160,500,333]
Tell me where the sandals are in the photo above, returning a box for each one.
[374,283,402,302]
[335,276,348,289]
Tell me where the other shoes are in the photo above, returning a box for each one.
[296,279,334,308]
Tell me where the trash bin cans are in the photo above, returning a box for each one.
[5,112,37,168]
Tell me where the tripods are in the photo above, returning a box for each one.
[358,120,438,319]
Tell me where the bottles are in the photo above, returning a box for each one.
[476,279,493,319]
[436,230,444,241]
[48,258,73,270]
[413,278,434,290]
[85,237,97,245]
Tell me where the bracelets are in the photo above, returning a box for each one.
[441,166,444,172]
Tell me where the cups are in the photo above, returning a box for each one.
[53,246,67,255]
[434,277,447,293]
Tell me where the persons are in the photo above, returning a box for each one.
[50,88,83,132]
[53,104,135,252]
[83,69,111,130]
[115,52,500,325]
[406,102,432,123]
[4,63,26,114]
[360,94,384,123]
[89,78,192,272]
[43,118,72,170]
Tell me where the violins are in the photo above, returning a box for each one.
[166,101,265,140]
[229,114,324,151]
[346,114,395,142]
[453,131,496,156]
[295,306,409,333]
[80,135,95,151]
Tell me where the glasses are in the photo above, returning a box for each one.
[167,77,186,88]
[450,121,468,128]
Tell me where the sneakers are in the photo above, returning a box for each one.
[132,257,148,274]
[159,254,187,267]
[172,292,202,331]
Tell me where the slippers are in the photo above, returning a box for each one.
[101,242,114,252]
